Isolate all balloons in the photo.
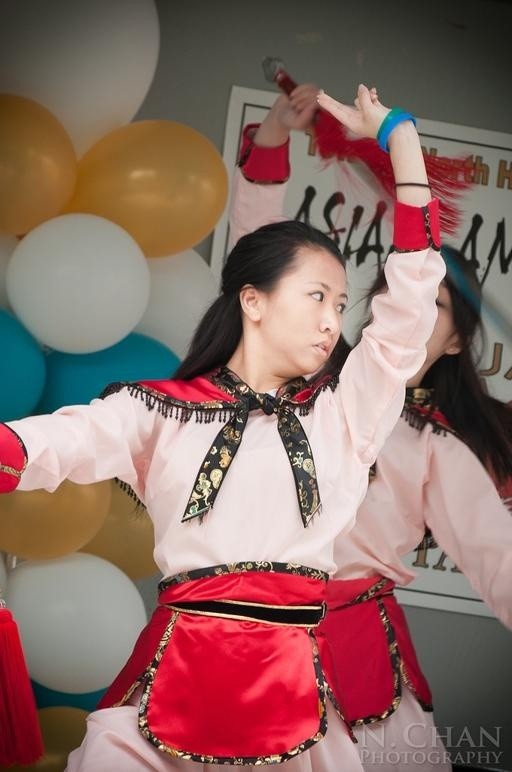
[0,1,228,772]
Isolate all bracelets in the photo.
[379,112,416,153]
[375,106,405,142]
[391,179,432,191]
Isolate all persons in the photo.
[0,81,449,772]
[225,81,512,772]
